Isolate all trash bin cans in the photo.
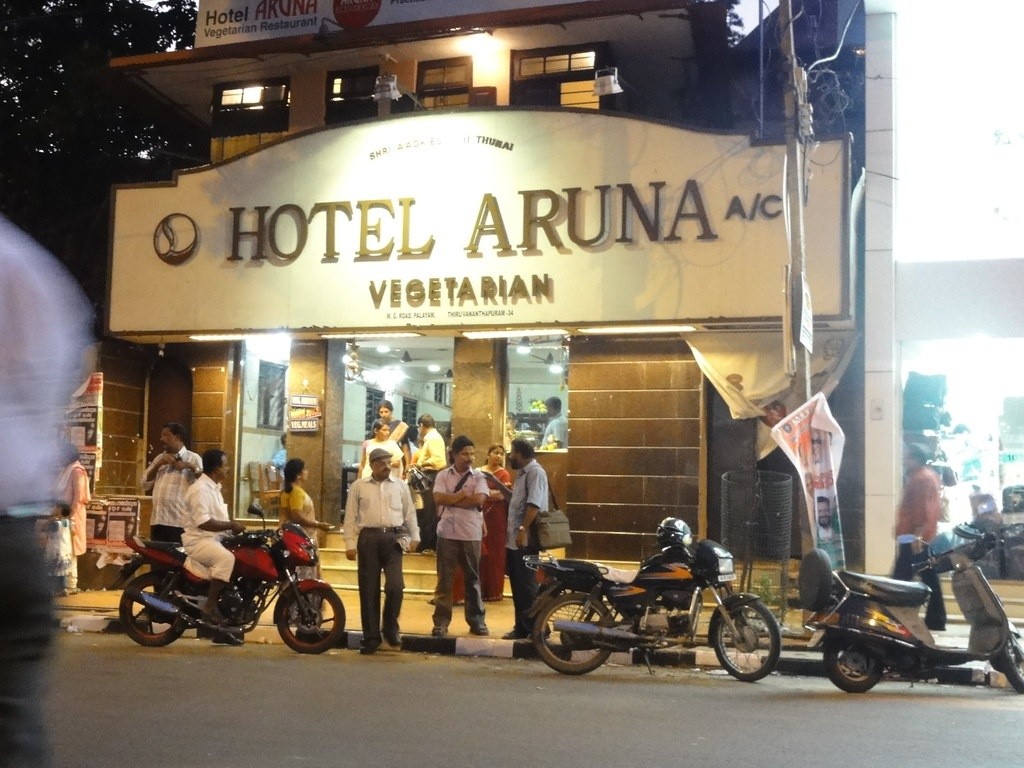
[973,521,1024,582]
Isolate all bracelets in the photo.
[316,521,318,526]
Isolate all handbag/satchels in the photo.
[525,465,572,550]
[425,515,442,551]
[408,467,430,492]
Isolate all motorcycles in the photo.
[522,524,783,682]
[108,504,347,655]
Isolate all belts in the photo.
[362,528,396,533]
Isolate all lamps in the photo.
[594,66,623,95]
[372,73,428,112]
[513,337,566,373]
[344,342,454,385]
[312,17,352,39]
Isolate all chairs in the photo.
[246,461,283,515]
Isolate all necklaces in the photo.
[500,484,506,492]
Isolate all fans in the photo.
[530,351,561,365]
[386,350,431,365]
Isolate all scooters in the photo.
[797,491,1024,694]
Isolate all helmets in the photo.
[656,517,692,547]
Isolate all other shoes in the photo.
[470,622,490,637]
[432,623,447,637]
[422,548,436,556]
[526,624,550,640]
[54,589,70,598]
[502,628,527,639]
[67,587,77,594]
[382,630,403,648]
[360,634,383,654]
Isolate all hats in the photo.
[370,448,393,465]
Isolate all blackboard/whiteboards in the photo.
[85,499,109,544]
[106,498,140,543]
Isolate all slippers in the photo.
[212,632,244,645]
[199,606,230,626]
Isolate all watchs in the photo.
[518,525,528,531]
[191,466,199,475]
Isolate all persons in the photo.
[271,433,287,485]
[521,419,534,431]
[508,412,518,432]
[892,443,947,632]
[181,448,249,649]
[432,434,490,636]
[539,396,569,449]
[356,400,423,485]
[0,214,93,768]
[344,448,422,656]
[536,419,542,432]
[817,495,832,540]
[481,439,548,640]
[140,420,204,593]
[474,442,513,601]
[50,442,91,597]
[37,501,76,598]
[278,458,335,635]
[408,413,448,557]
